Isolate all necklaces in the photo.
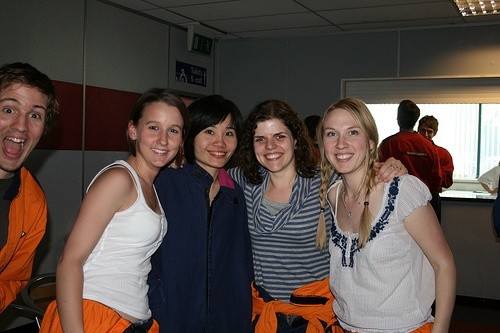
[340,189,367,219]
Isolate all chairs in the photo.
[20,273,56,313]
[0,305,49,333]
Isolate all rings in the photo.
[391,165,395,170]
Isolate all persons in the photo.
[311,95,458,333]
[158,101,411,333]
[375,97,442,224]
[145,92,257,333]
[35,86,192,333]
[414,113,455,224]
[1,60,64,325]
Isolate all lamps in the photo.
[452,0,500,18]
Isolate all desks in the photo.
[435,190,500,306]
